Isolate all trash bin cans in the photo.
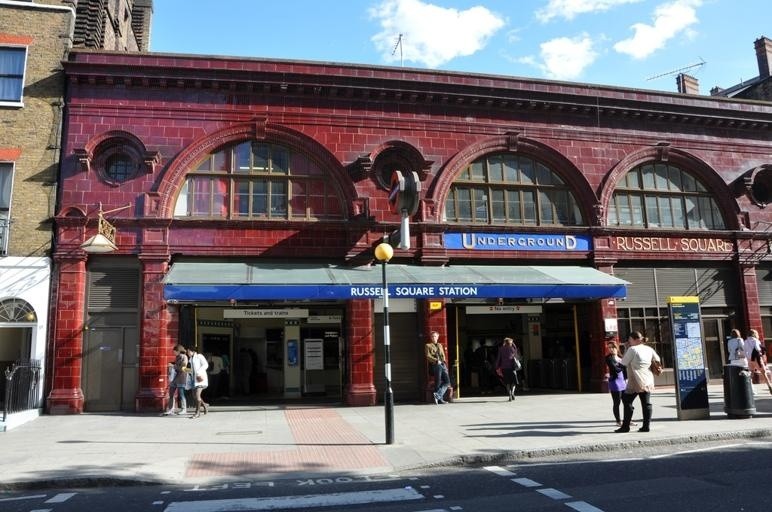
[724,365,756,419]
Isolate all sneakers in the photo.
[433,392,448,404]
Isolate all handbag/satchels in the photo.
[652,358,663,375]
[735,338,745,359]
[513,357,522,371]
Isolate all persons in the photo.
[744,329,772,395]
[605,342,638,426]
[495,337,519,401]
[728,328,756,395]
[163,344,257,419]
[610,331,661,433]
[425,331,450,404]
[472,340,496,394]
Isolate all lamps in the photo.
[77,201,133,253]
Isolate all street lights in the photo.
[374,243,394,444]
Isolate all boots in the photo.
[614,406,638,433]
[639,405,652,432]
[162,398,175,415]
[507,384,515,401]
[190,400,209,419]
[177,399,187,415]
